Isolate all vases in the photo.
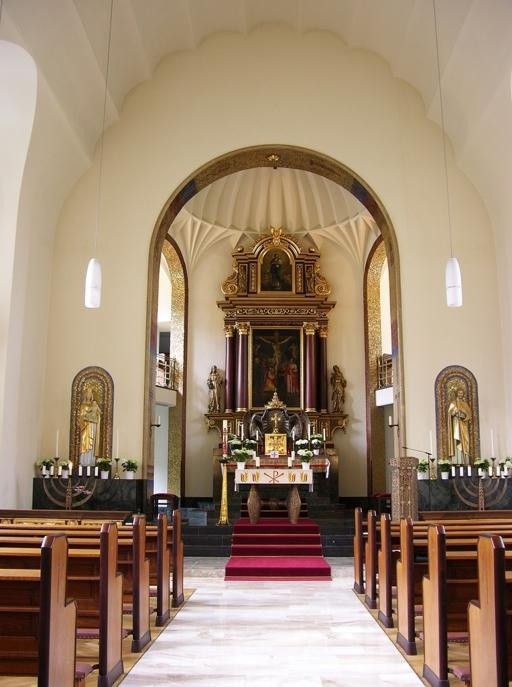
[101,471,108,479]
[44,470,50,478]
[61,470,69,479]
[246,450,254,455]
[481,469,488,477]
[236,462,246,469]
[314,449,320,456]
[298,449,305,456]
[231,450,236,455]
[418,472,427,480]
[441,472,449,480]
[301,462,310,470]
[125,471,134,479]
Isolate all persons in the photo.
[77,390,102,465]
[206,364,224,412]
[329,365,347,412]
[446,387,475,467]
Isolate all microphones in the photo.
[402,447,409,449]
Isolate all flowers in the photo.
[474,457,490,472]
[245,439,257,450]
[297,448,313,463]
[121,457,139,472]
[233,448,248,462]
[310,433,324,449]
[416,456,429,472]
[228,439,242,450]
[438,458,451,472]
[96,457,113,471]
[57,456,71,470]
[295,439,308,449]
[36,456,56,470]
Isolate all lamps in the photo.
[433,0,463,307]
[84,0,113,308]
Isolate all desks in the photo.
[227,460,326,518]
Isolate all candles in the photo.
[490,429,495,458]
[291,451,295,461]
[157,416,160,424]
[496,467,501,476]
[241,424,243,441]
[58,466,62,475]
[323,429,326,441]
[478,468,481,476]
[238,422,240,436]
[79,465,83,476]
[452,466,455,477]
[256,457,260,467]
[256,430,258,440]
[69,463,73,475]
[467,466,471,476]
[87,466,91,476]
[459,467,464,476]
[287,457,292,467]
[389,416,391,425]
[292,429,294,440]
[95,467,98,476]
[42,466,46,475]
[504,466,508,475]
[307,424,310,440]
[430,430,432,458]
[50,466,55,475]
[252,451,256,460]
[488,466,493,476]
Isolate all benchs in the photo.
[0,510,185,687]
[351,507,512,687]
